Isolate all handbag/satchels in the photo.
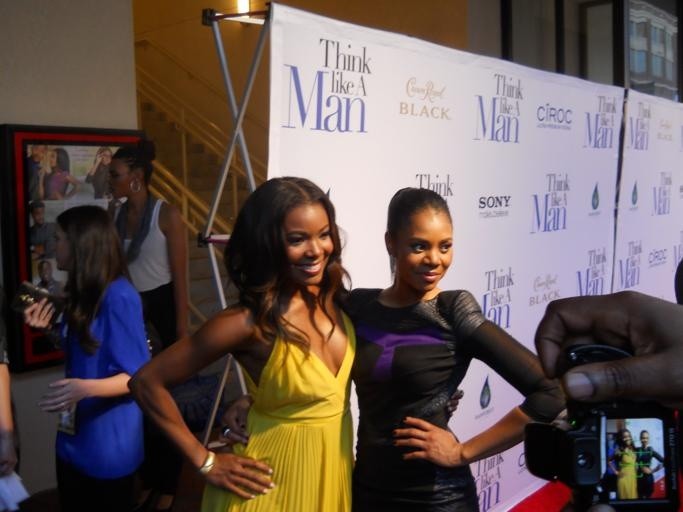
[171,375,225,429]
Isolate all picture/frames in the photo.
[1,124,145,374]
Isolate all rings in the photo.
[222,425,231,436]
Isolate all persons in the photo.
[104,138,189,510]
[127,178,358,511]
[0,313,32,512]
[607,439,619,490]
[534,286,682,512]
[22,204,155,512]
[608,428,640,499]
[635,430,664,498]
[216,187,565,512]
[27,143,113,302]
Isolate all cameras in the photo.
[525,343,679,512]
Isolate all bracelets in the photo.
[196,448,216,475]
